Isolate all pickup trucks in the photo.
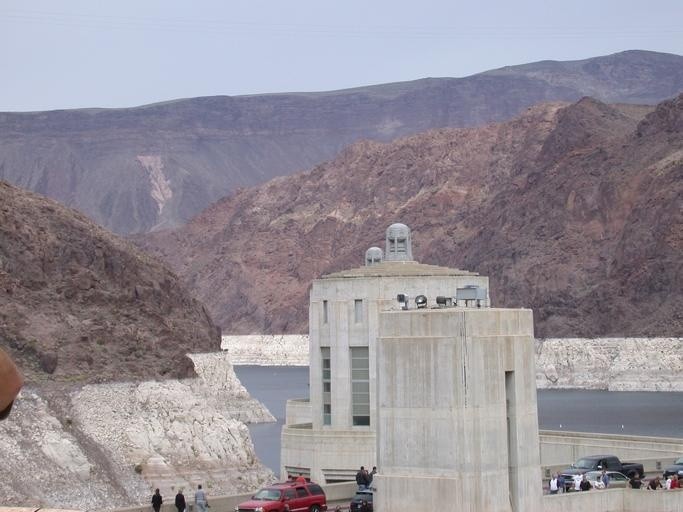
[556,454,646,490]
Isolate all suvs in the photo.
[232,481,328,512]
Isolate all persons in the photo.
[355,466,368,489]
[193,485,211,512]
[174,489,187,512]
[367,466,377,482]
[152,488,163,512]
[647,476,663,490]
[548,471,593,495]
[294,472,307,482]
[594,476,606,490]
[662,473,683,490]
[601,469,610,488]
[286,475,292,482]
[625,472,646,490]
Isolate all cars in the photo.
[348,489,374,511]
[662,456,682,483]
[566,471,641,490]
